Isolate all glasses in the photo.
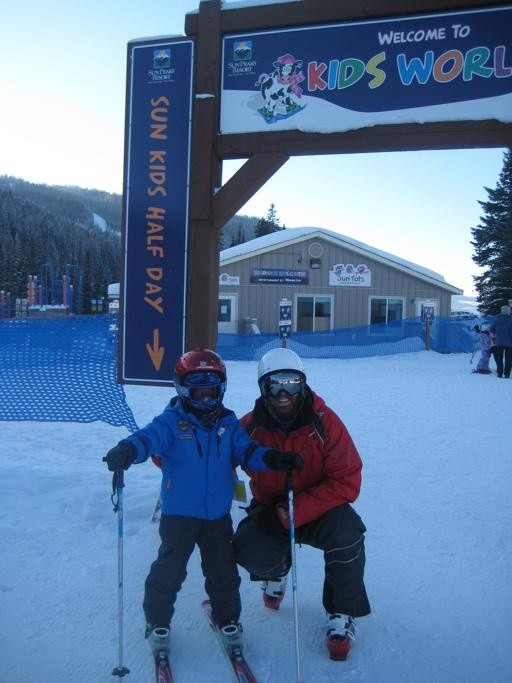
[181,372,224,387]
[263,383,301,397]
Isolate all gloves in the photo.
[264,449,305,473]
[255,508,286,537]
[105,442,137,472]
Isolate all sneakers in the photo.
[260,574,288,598]
[325,612,357,645]
[143,622,172,653]
[211,618,244,648]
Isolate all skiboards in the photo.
[144,600,257,683]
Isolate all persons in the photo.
[468,305,511,378]
[105,344,305,665]
[226,345,367,656]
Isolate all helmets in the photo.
[172,347,228,420]
[256,347,310,429]
